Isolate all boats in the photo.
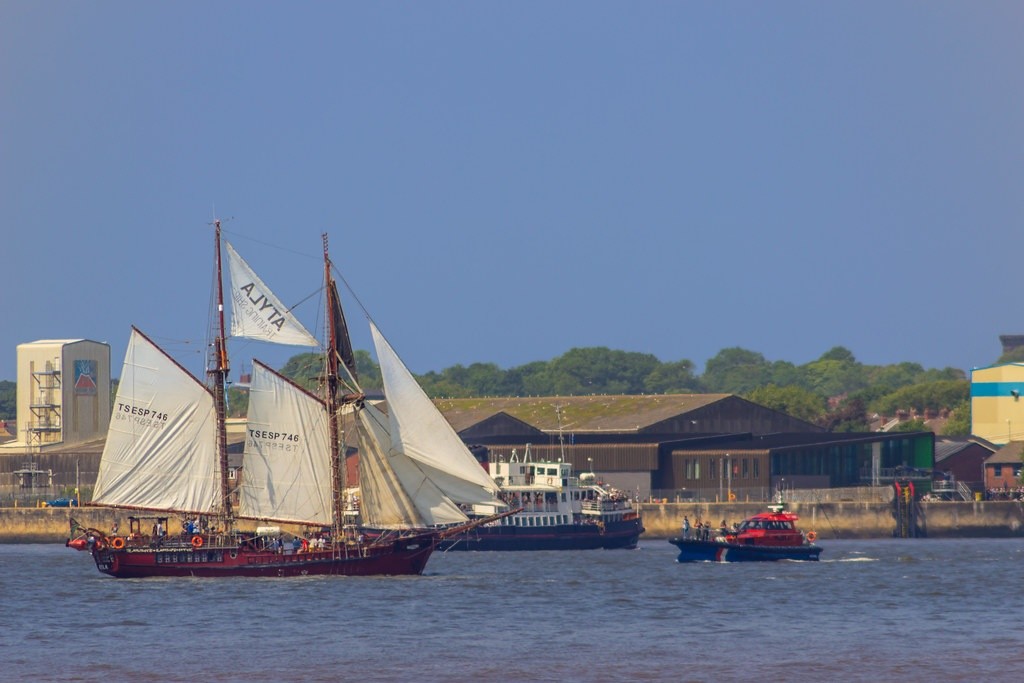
[667,475,824,565]
[341,403,646,553]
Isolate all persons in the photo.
[694,520,711,541]
[985,486,1024,500]
[332,531,364,545]
[181,518,196,536]
[276,533,286,556]
[302,537,307,552]
[308,534,326,553]
[682,515,691,538]
[741,520,786,530]
[718,519,738,540]
[203,519,217,534]
[110,522,120,538]
[152,520,165,550]
[489,469,631,525]
[292,535,300,551]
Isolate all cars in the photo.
[46,497,78,507]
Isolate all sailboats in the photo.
[64,215,526,580]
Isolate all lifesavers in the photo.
[547,478,553,485]
[112,537,125,549]
[806,531,817,542]
[192,536,203,547]
[729,494,736,500]
